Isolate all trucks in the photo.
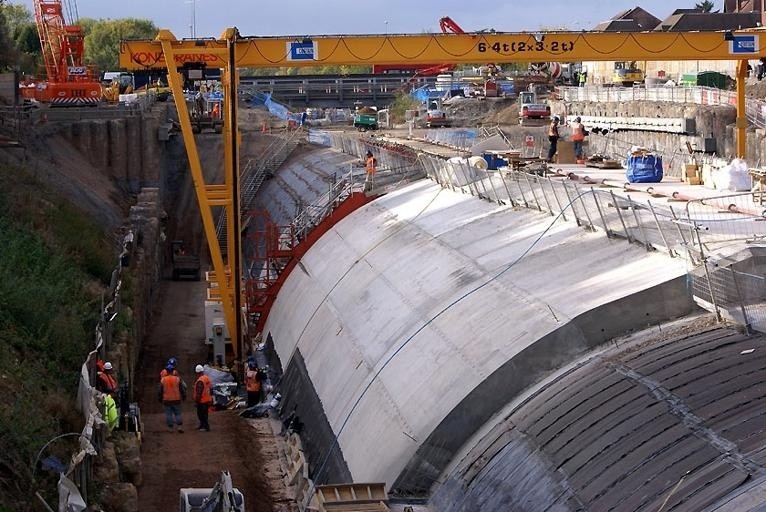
[353,113,378,131]
[170,237,202,281]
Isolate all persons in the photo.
[572,117,585,162]
[96,358,212,432]
[211,104,219,121]
[757,60,764,81]
[579,73,586,87]
[747,62,752,78]
[196,93,206,116]
[546,117,560,162]
[363,150,377,192]
[244,357,258,385]
[246,361,267,407]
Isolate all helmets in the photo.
[248,361,258,369]
[103,362,112,369]
[195,365,204,372]
[166,358,177,370]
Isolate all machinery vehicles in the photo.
[197,267,240,372]
[602,60,645,88]
[190,89,225,135]
[525,61,574,89]
[15,1,104,110]
[181,471,245,512]
[517,92,552,130]
[414,94,456,128]
[105,77,173,105]
